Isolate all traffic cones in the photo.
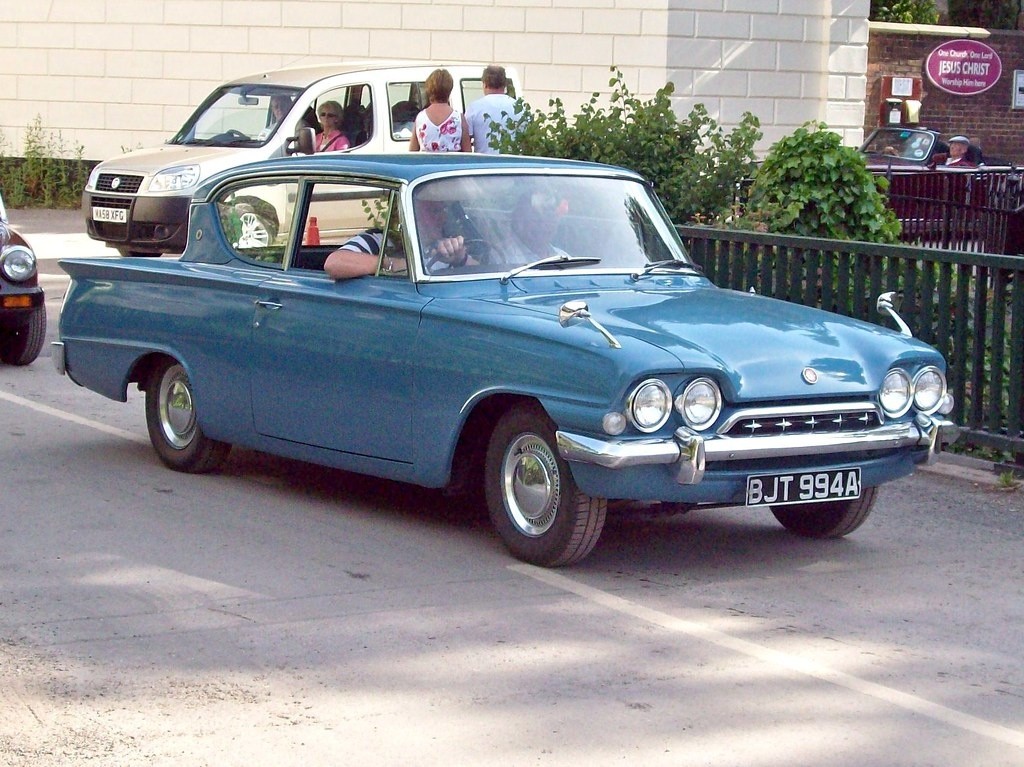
[305,216,321,246]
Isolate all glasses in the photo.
[320,113,336,117]
[429,205,449,217]
[272,103,286,108]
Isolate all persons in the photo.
[944,135,980,167]
[409,68,472,152]
[322,192,482,280]
[885,126,946,163]
[465,63,527,155]
[255,95,293,142]
[485,185,575,266]
[311,100,351,153]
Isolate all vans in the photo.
[81,56,524,257]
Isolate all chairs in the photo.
[302,106,323,135]
[337,106,368,149]
[928,141,952,165]
[965,145,983,167]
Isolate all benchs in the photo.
[233,245,345,271]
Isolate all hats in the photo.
[948,135,970,145]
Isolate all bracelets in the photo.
[387,257,394,272]
[453,253,469,268]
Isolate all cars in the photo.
[49,153,960,569]
[0,190,47,365]
[723,124,1024,231]
